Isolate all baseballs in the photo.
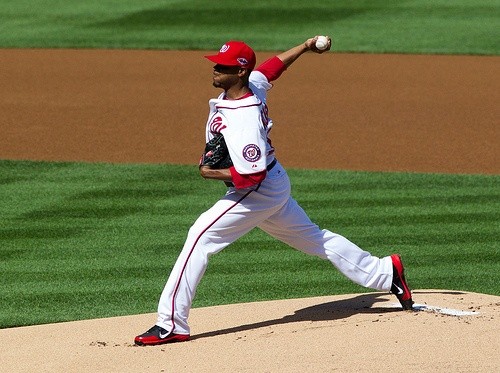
[315,35,328,50]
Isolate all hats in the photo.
[204,40,256,69]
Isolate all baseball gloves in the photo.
[198,134,233,188]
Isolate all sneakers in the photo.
[134,326,190,345]
[391,254,414,310]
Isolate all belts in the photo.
[267,158,277,172]
